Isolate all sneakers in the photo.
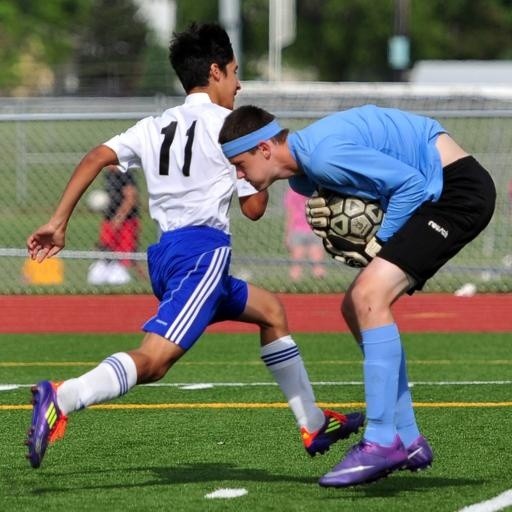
[301,410,433,487]
[26,380,66,467]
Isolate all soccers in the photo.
[325,196,384,250]
[86,190,111,213]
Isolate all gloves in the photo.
[305,190,332,238]
[322,236,382,267]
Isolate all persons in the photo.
[87,164,141,286]
[218,104,495,487]
[20,256,63,286]
[283,188,324,280]
[26,22,366,469]
